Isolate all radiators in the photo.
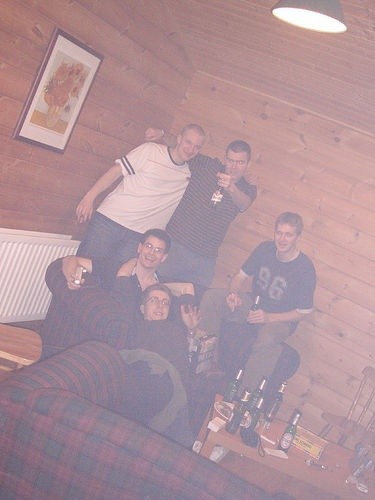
[0,228,81,322]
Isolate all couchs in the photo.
[44,256,301,407]
[0,340,290,500]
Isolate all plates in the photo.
[214,401,251,426]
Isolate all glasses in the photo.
[146,296,170,306]
[142,243,163,255]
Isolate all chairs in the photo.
[0,323,44,368]
[319,366,375,452]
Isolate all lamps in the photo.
[272,0,348,34]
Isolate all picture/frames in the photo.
[14,27,103,152]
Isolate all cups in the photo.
[72,264,87,286]
[351,459,373,477]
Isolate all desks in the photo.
[199,391,375,500]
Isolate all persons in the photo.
[75,122,257,306]
[61,229,202,449]
[198,213,316,401]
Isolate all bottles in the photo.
[247,296,260,325]
[225,389,252,434]
[263,382,288,421]
[240,394,265,437]
[277,413,301,454]
[207,186,224,210]
[242,377,267,414]
[223,368,244,403]
